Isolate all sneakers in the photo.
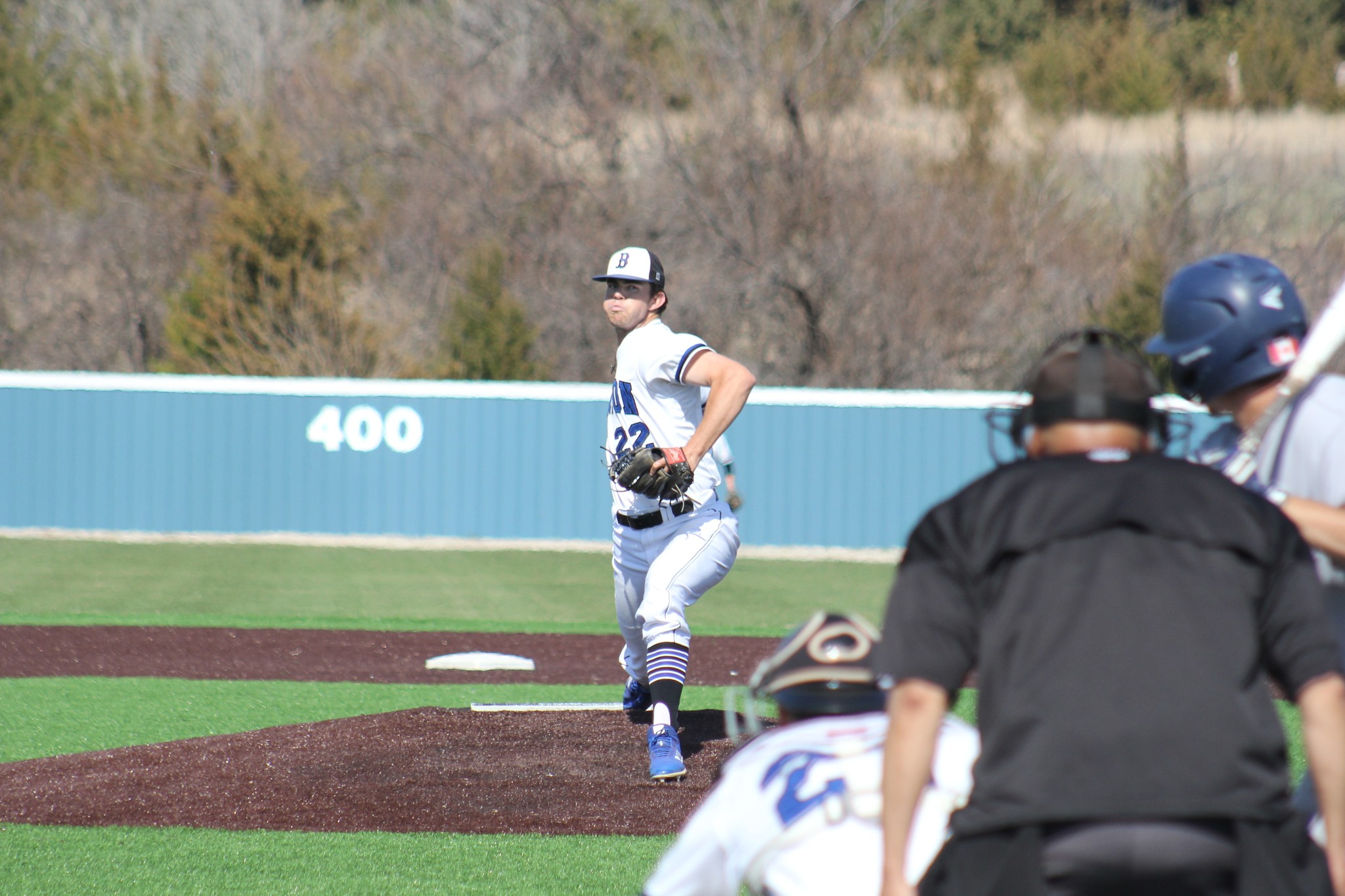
[647,723,686,782]
[623,676,652,714]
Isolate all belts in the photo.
[617,499,694,530]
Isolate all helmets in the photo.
[751,614,883,714]
[1142,254,1307,399]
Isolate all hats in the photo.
[592,246,665,286]
[1010,350,1151,445]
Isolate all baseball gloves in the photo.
[607,438,694,498]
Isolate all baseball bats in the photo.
[1241,277,1345,459]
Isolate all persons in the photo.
[1142,254,1345,854]
[642,614,980,896]
[593,247,756,782]
[874,330,1345,896]
